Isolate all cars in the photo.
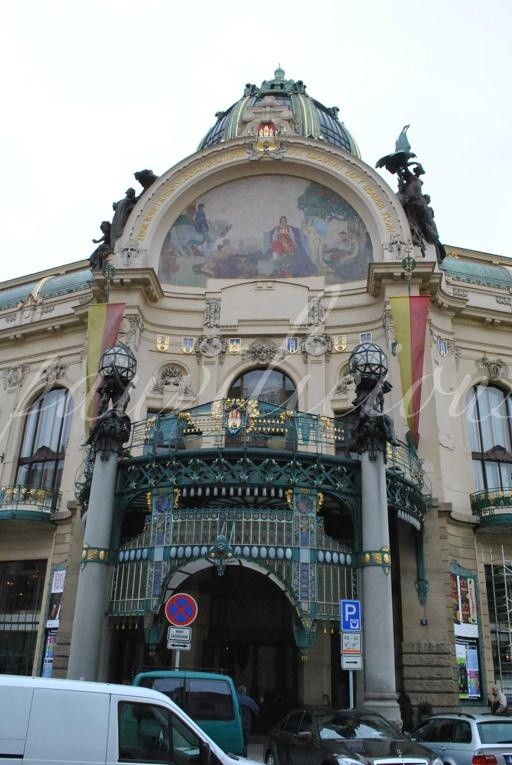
[405,711,512,765]
[263,704,445,764]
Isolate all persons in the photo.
[237,685,260,750]
[491,685,508,714]
[192,201,374,282]
[395,689,414,735]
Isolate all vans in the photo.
[0,673,268,765]
[119,670,246,759]
